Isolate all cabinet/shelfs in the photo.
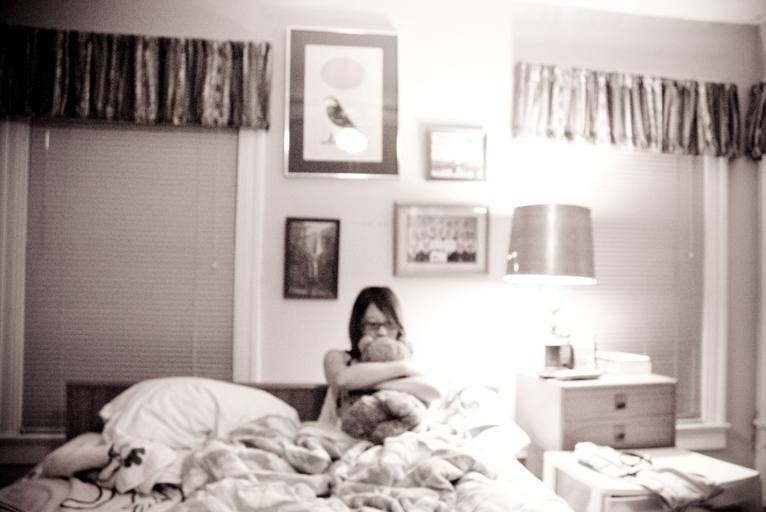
[541,447,763,511]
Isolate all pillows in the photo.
[99,375,298,443]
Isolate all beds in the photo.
[0,383,574,512]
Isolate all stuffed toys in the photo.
[339,333,426,439]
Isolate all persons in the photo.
[315,286,444,452]
[402,218,479,268]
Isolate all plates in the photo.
[536,367,602,379]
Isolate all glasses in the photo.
[366,321,398,330]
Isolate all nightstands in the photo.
[519,368,677,450]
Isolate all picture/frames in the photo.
[283,216,341,301]
[394,201,490,280]
[284,25,400,178]
[427,127,487,181]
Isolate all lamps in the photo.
[503,203,604,380]
